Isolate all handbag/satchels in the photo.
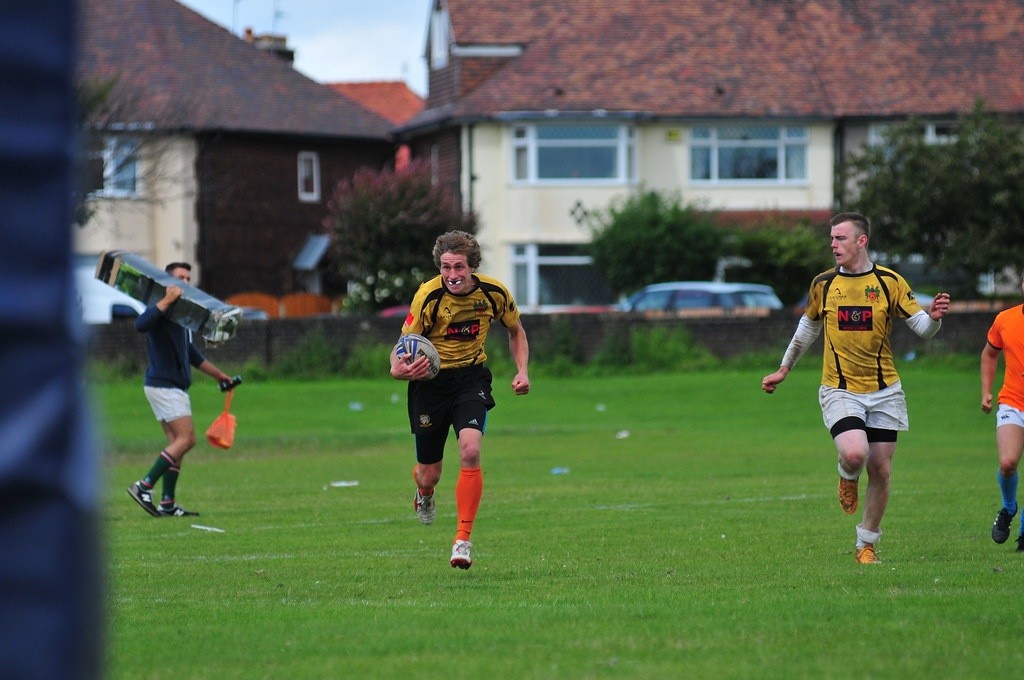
[206,388,237,449]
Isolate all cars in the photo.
[73,261,151,325]
[612,280,785,316]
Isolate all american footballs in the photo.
[397,333,441,381]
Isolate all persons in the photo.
[389,231,529,569]
[127,262,233,518]
[981,272,1024,553]
[762,212,950,563]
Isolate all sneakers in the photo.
[126,480,161,517]
[1015,531,1024,553]
[413,488,437,525]
[856,544,881,565]
[992,501,1018,544]
[450,536,473,570]
[838,475,859,515]
[157,504,199,517]
